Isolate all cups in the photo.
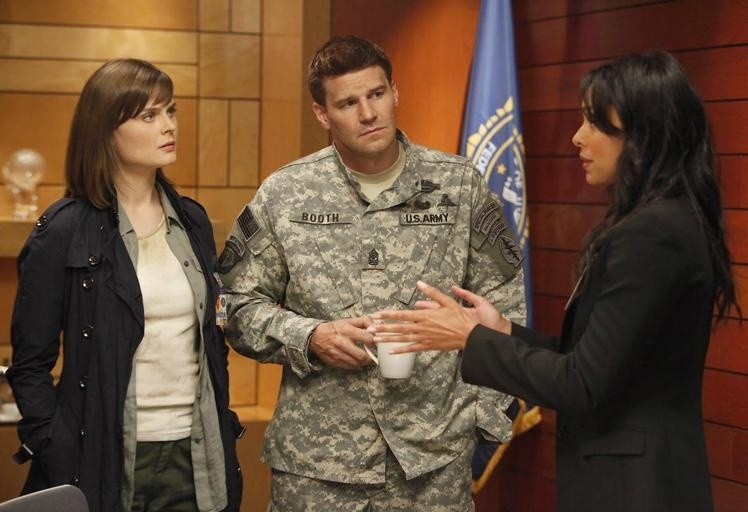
[364,332,415,380]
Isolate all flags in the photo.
[460,0,542,494]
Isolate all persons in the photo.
[5,58,247,512]
[367,50,743,511]
[213,36,528,512]
